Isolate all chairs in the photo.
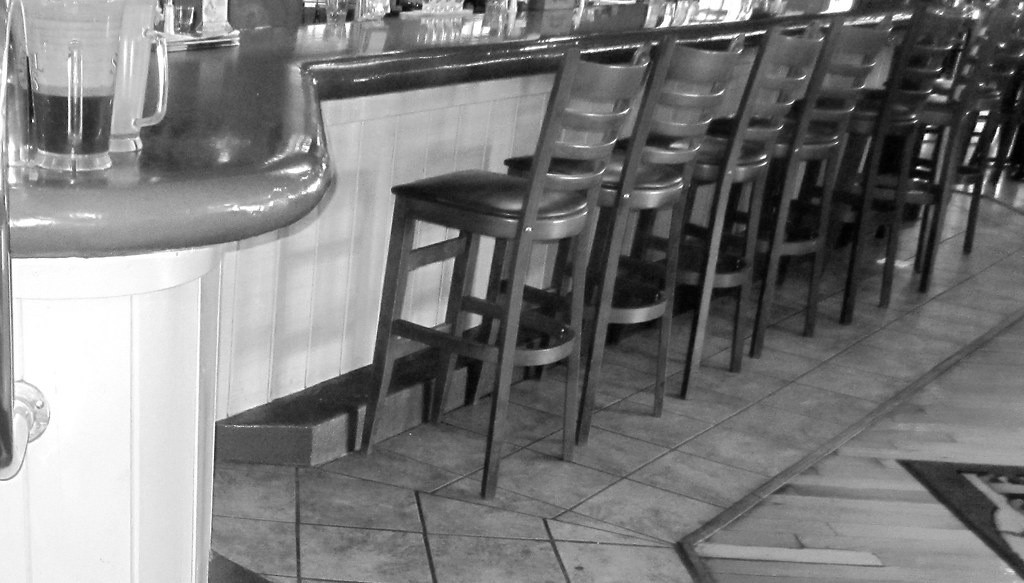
[362,0,1024,499]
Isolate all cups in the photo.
[643,0,788,30]
[0,0,195,172]
[323,1,530,31]
